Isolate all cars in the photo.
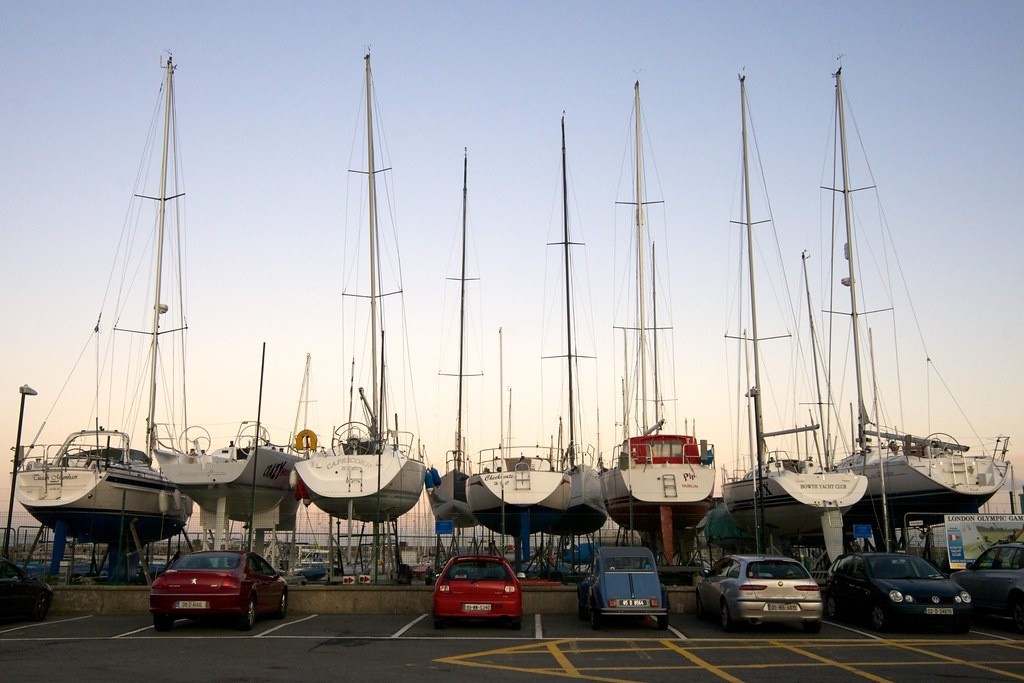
[0,556,52,626]
[149,550,287,631]
[698,553,823,635]
[430,556,523,629]
[580,547,670,632]
[827,552,973,634]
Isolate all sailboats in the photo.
[26,54,199,546]
[810,58,1011,532]
[421,145,497,529]
[535,112,606,540]
[700,74,872,544]
[305,45,419,527]
[598,76,718,536]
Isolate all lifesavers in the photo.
[296,429,318,451]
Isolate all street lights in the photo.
[3,384,37,559]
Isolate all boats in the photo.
[467,440,575,538]
[152,414,309,519]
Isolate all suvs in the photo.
[952,541,1024,635]
